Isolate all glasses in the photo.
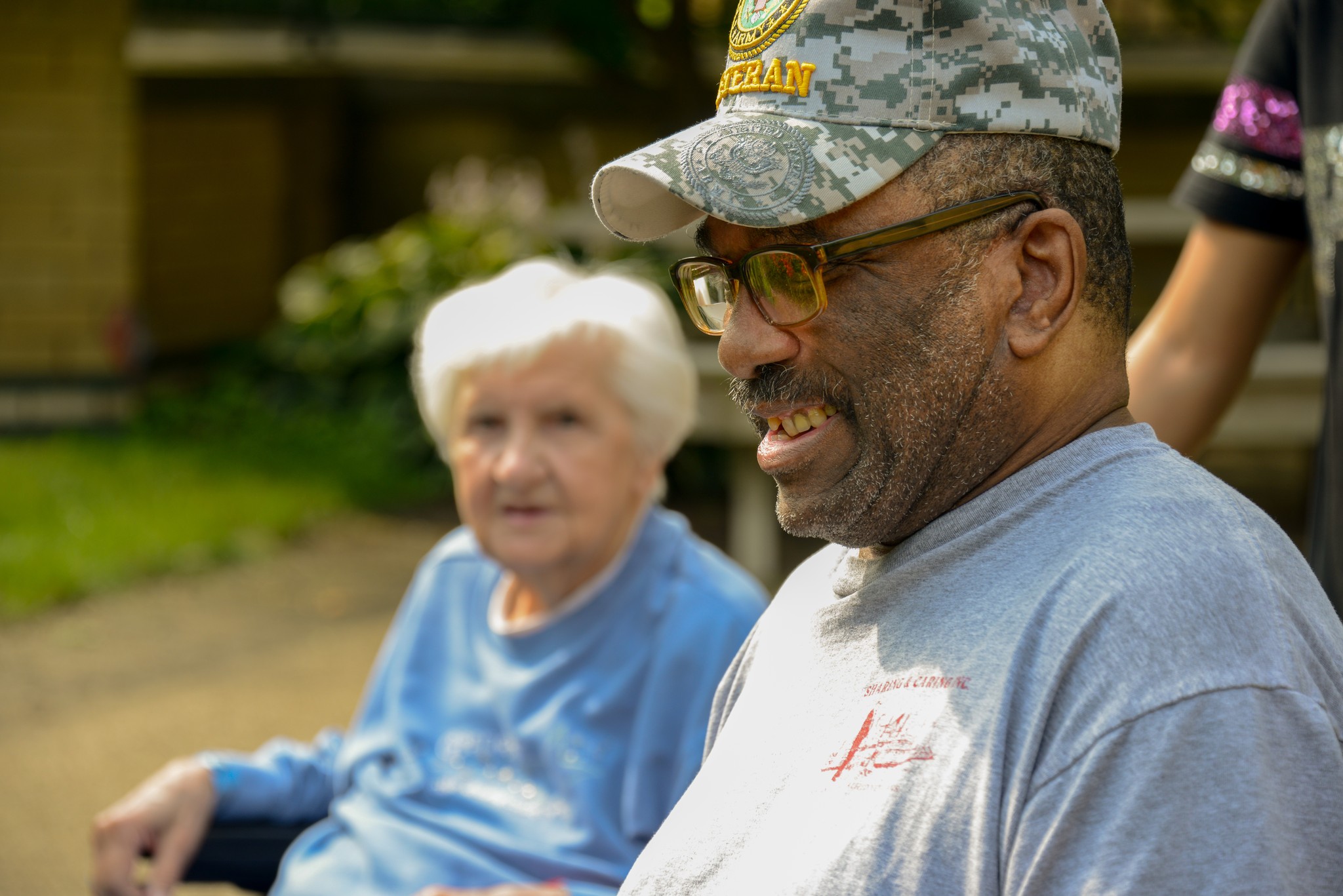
[669,190,1047,336]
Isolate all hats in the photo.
[588,0,1123,245]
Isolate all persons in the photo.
[89,255,770,896]
[1125,0,1343,624]
[590,1,1343,896]
